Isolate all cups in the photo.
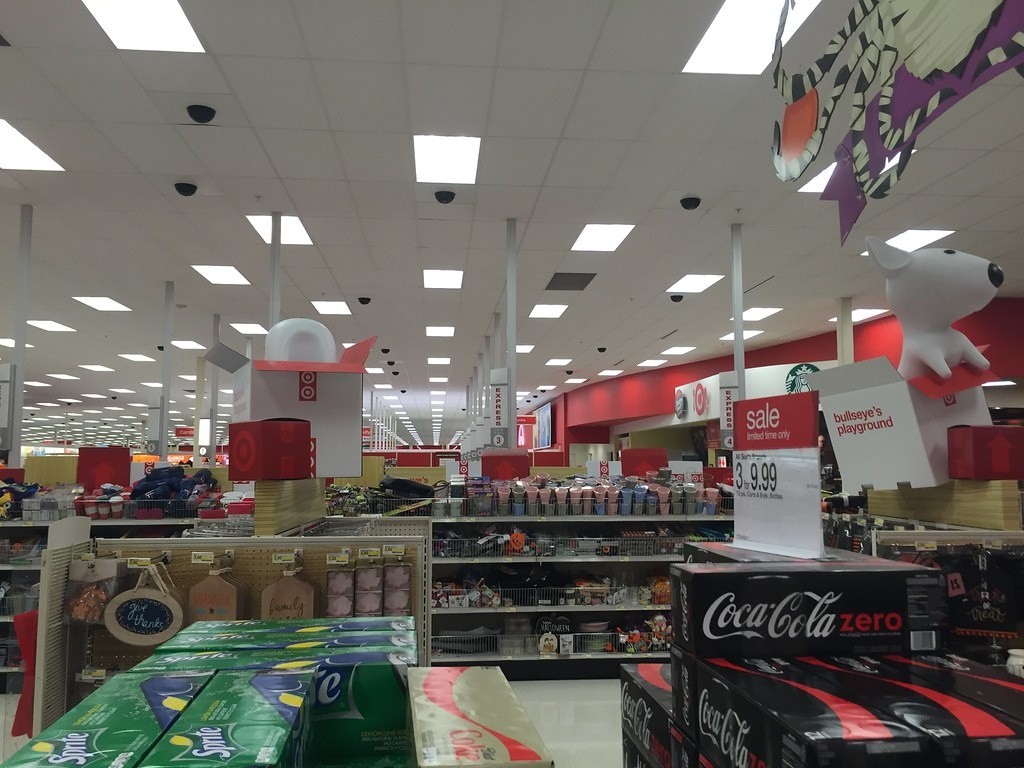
[494,466,722,517]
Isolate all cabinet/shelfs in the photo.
[0,477,736,696]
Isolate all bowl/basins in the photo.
[577,620,611,632]
[580,638,613,651]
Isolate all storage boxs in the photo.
[0,669,218,768]
[683,542,820,564]
[793,666,1024,768]
[620,663,673,768]
[225,417,311,482]
[947,424,1024,481]
[885,652,1024,722]
[126,646,417,759]
[671,565,948,658]
[816,546,897,564]
[694,661,931,768]
[136,669,316,768]
[405,666,556,768]
[622,728,653,768]
[802,343,1001,496]
[177,615,415,635]
[202,335,377,479]
[155,630,418,653]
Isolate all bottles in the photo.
[1006,649,1024,678]
